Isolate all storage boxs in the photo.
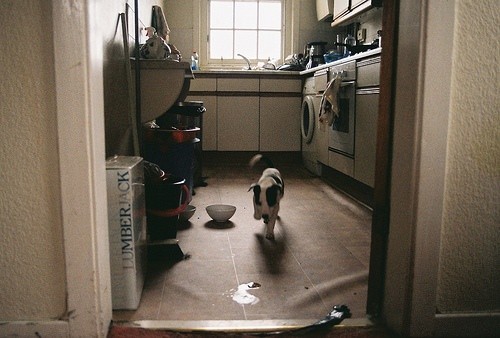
[106,155,151,313]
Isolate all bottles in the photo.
[191,51,199,71]
[337,33,344,52]
[343,34,356,57]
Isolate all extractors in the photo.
[330,1,384,28]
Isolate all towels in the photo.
[317,74,341,126]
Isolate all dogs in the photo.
[247,168,284,239]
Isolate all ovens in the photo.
[322,60,356,196]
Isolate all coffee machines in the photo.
[304,41,327,67]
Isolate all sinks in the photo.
[205,66,246,72]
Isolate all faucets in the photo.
[237,53,252,70]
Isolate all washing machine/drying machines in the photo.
[299,77,322,176]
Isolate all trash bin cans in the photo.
[175,101,209,187]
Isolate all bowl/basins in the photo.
[170,125,199,143]
[179,205,196,224]
[323,52,342,63]
[206,204,236,223]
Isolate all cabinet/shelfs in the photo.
[330,0,384,30]
[188,54,380,211]
